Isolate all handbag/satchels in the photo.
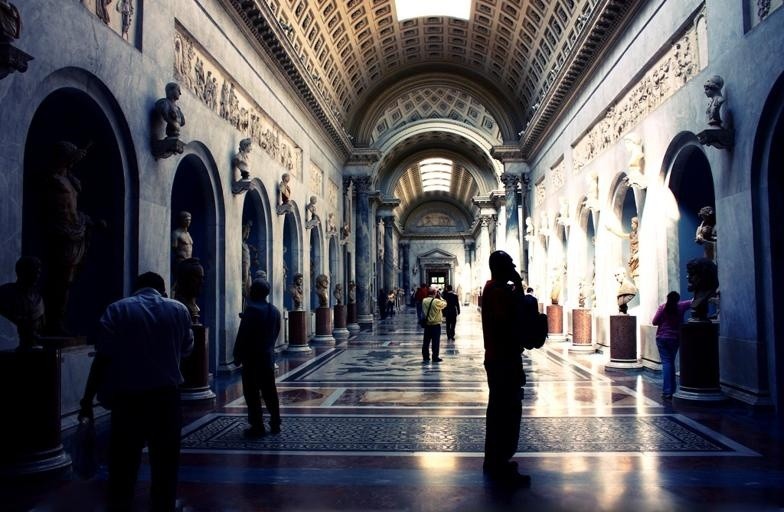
[421,312,427,327]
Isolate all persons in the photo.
[376,278,462,365]
[155,81,186,146]
[236,138,349,245]
[700,73,728,131]
[481,249,548,489]
[229,279,282,441]
[46,136,106,328]
[172,209,203,324]
[242,219,260,297]
[611,267,637,311]
[603,216,637,277]
[683,257,719,317]
[651,292,698,401]
[172,34,295,170]
[687,202,715,244]
[288,271,357,310]
[3,256,46,357]
[78,272,194,510]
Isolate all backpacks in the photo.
[523,295,548,350]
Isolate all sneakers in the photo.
[270,423,281,434]
[433,358,443,362]
[509,462,518,468]
[519,474,532,483]
[244,424,266,437]
